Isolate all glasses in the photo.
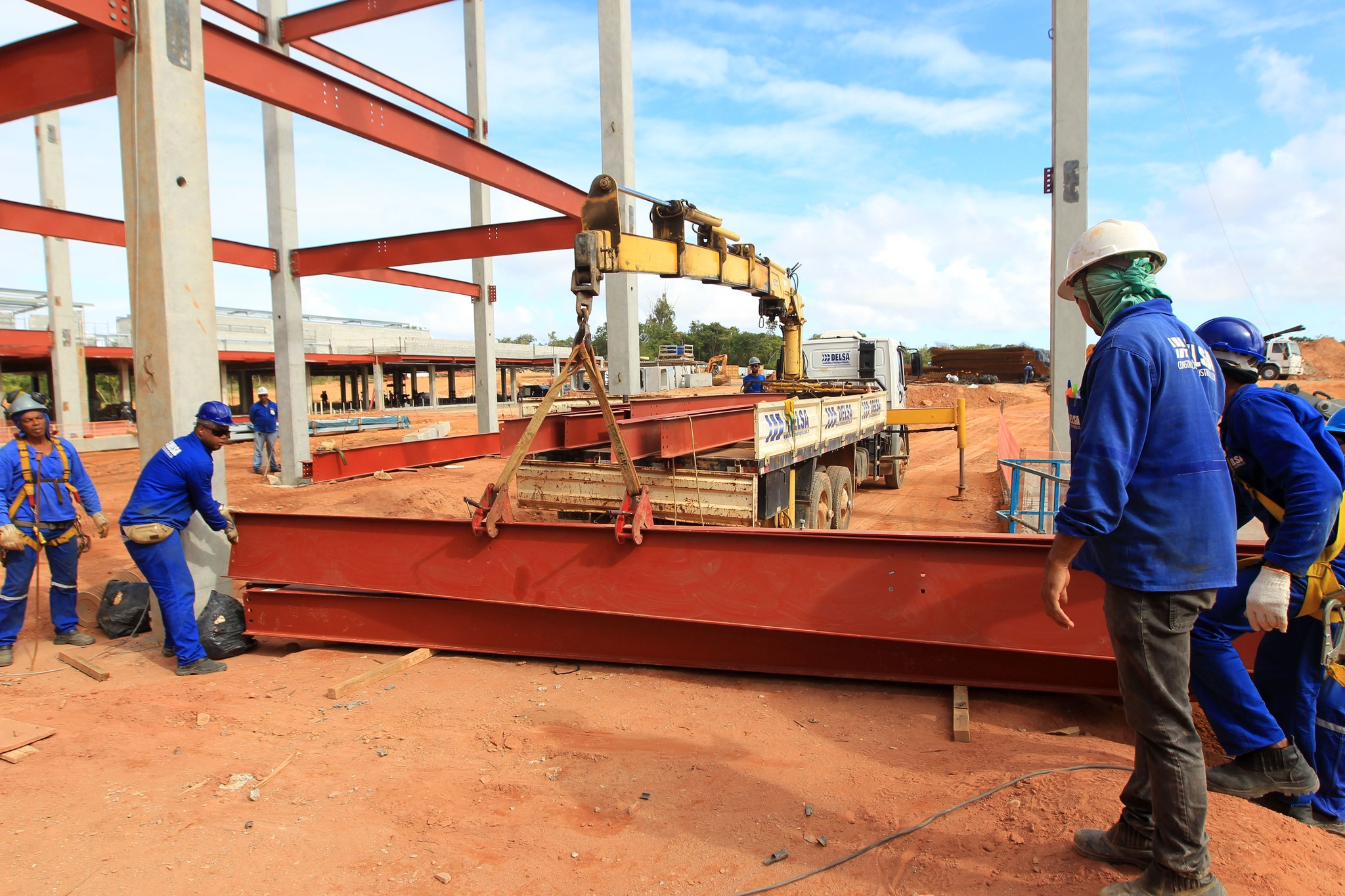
[751,365,759,368]
[203,426,231,438]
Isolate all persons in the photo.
[117,401,238,675]
[320,391,328,406]
[1023,366,1034,384]
[743,357,772,393]
[403,394,410,399]
[1041,220,1238,896]
[249,386,281,473]
[384,390,393,407]
[0,389,109,666]
[1189,317,1345,834]
[419,392,424,399]
[361,392,363,400]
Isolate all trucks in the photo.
[1256,338,1304,381]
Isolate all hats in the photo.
[1214,349,1259,386]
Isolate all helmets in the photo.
[4,392,50,419]
[1056,217,1167,301]
[749,356,761,365]
[194,400,239,426]
[1194,317,1266,362]
[256,386,268,394]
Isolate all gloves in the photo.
[217,503,234,524]
[1243,565,1291,633]
[91,510,108,539]
[222,519,238,545]
[0,524,24,552]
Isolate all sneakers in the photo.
[1206,734,1320,800]
[162,646,176,657]
[1099,866,1228,896]
[1074,826,1152,870]
[254,467,264,474]
[270,468,282,472]
[53,629,95,646]
[0,645,13,666]
[1308,809,1345,836]
[1251,794,1313,823]
[176,656,227,676]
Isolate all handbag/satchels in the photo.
[121,522,174,544]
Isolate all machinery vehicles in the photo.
[452,173,972,541]
